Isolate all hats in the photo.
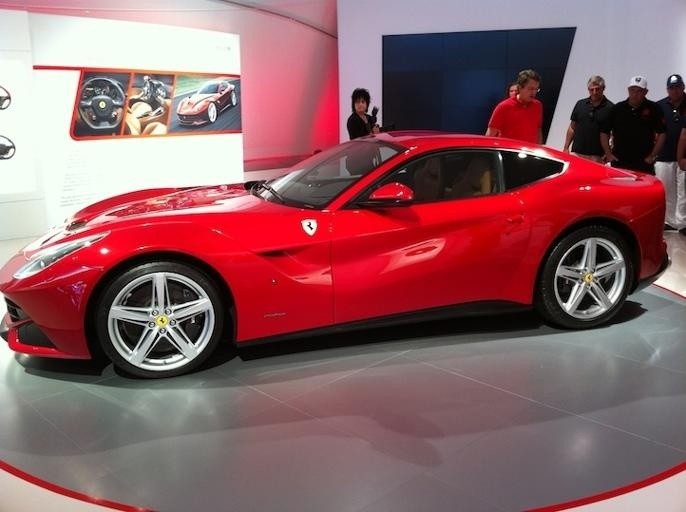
[628,76,649,91]
[666,74,683,88]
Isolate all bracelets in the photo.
[604,149,612,154]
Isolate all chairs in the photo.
[412,154,493,203]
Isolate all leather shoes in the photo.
[663,223,678,230]
[679,227,685,238]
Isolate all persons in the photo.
[505,81,519,99]
[344,87,384,175]
[656,74,686,231]
[597,76,667,185]
[562,75,615,166]
[481,69,544,145]
[674,124,686,238]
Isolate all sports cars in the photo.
[176,79,237,126]
[0,131,672,379]
